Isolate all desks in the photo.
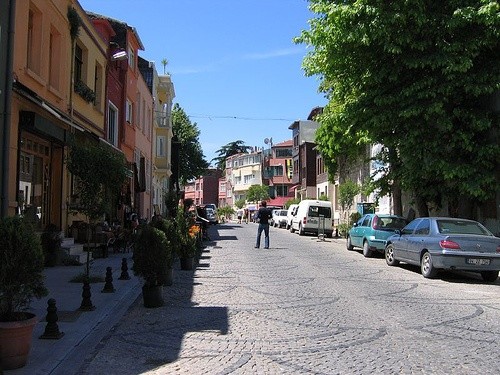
[94,231,127,258]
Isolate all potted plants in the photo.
[0,215,49,370]
[157,207,202,286]
[134,225,170,308]
[40,224,63,266]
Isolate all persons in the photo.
[253,201,273,249]
[237,208,243,224]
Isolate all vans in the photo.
[288,200,334,237]
[203,203,220,227]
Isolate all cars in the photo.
[272,204,299,229]
[384,216,500,282]
[345,213,410,257]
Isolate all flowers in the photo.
[188,225,201,239]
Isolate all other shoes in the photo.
[254,246,259,249]
[264,247,269,249]
